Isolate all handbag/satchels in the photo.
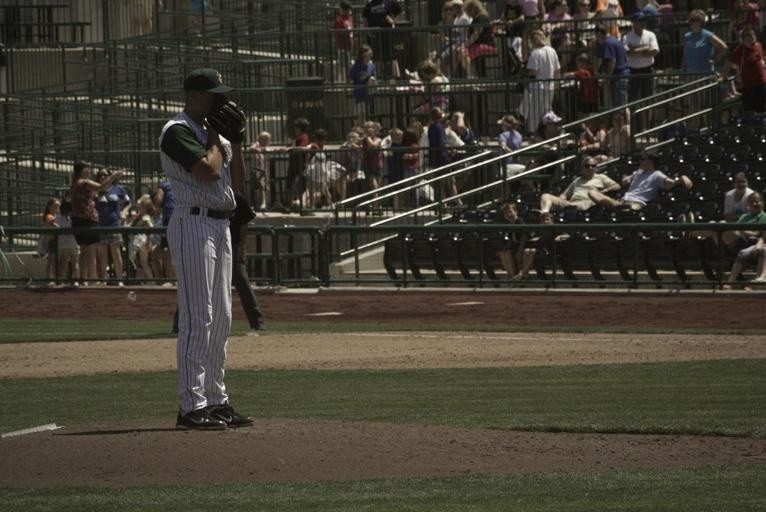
[417,179,435,202]
[59,198,75,213]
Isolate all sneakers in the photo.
[209,403,253,426]
[173,409,225,431]
[246,316,268,333]
[43,276,179,291]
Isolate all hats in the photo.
[425,105,443,119]
[72,160,90,169]
[180,68,234,94]
[494,110,562,128]
[631,11,647,21]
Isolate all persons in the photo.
[170,190,268,333]
[40,159,180,290]
[233,2,765,292]
[157,68,254,431]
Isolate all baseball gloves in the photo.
[207,96,247,143]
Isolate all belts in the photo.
[187,205,233,220]
